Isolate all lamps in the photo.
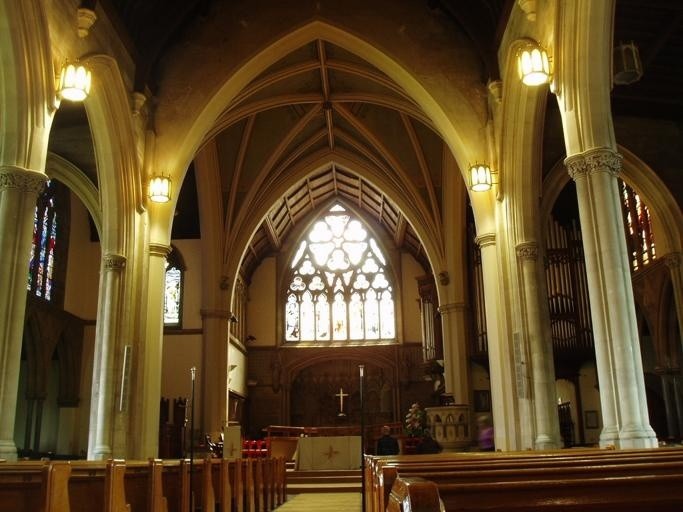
[516,46,554,87]
[148,172,172,203]
[614,40,642,87]
[468,160,498,193]
[56,58,94,104]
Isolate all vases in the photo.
[399,435,427,449]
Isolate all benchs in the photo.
[0,445,289,512]
[362,446,683,512]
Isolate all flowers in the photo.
[406,403,427,435]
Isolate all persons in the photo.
[473,414,495,452]
[375,424,401,456]
[413,432,443,453]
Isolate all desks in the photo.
[294,436,363,470]
